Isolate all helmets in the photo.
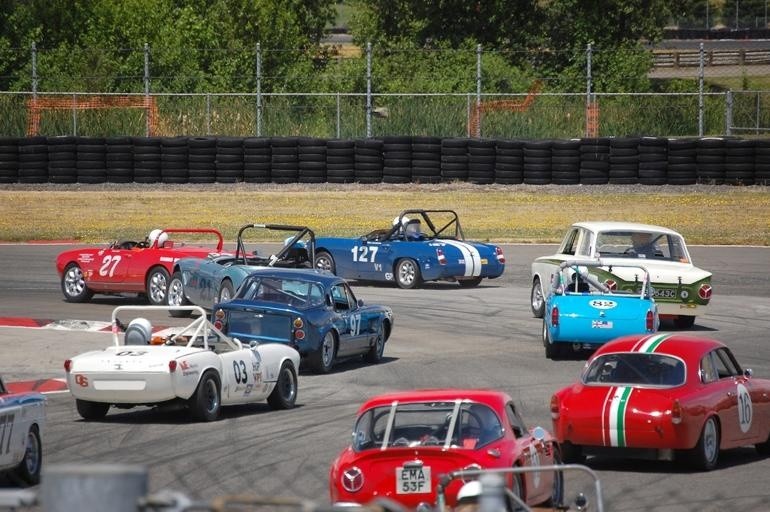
[567,265,587,284]
[392,216,411,236]
[127,318,152,341]
[284,236,304,249]
[466,406,497,432]
[631,232,654,249]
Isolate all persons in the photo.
[392,216,410,232]
[128,317,152,345]
[269,237,305,261]
[568,273,589,292]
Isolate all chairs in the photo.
[280,248,309,260]
[468,404,499,431]
[568,282,589,292]
